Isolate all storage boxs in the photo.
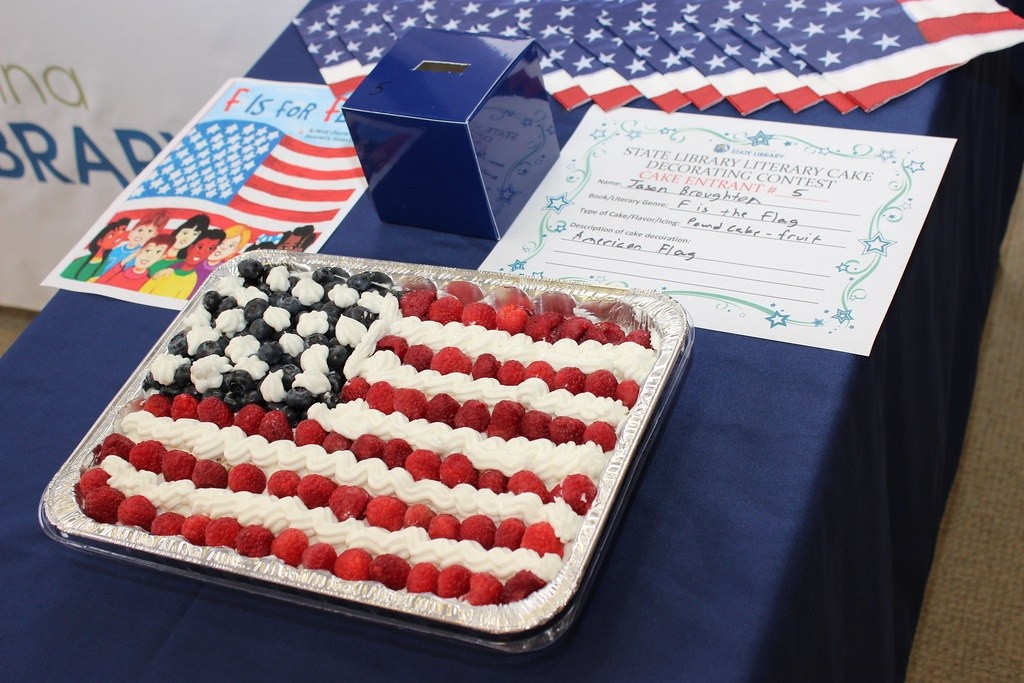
[341,29,562,240]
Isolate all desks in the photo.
[2,0,1023,683]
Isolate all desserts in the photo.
[80,257,657,604]
